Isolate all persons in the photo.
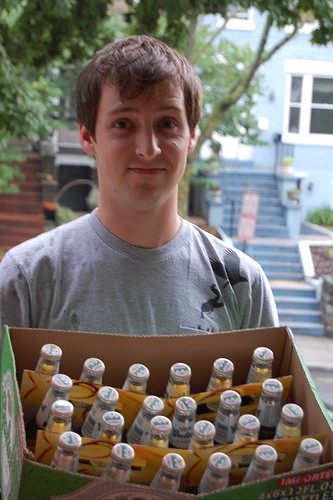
[0,35,280,347]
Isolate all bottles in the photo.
[32,343,324,493]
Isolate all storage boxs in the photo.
[0,325,333,500]
